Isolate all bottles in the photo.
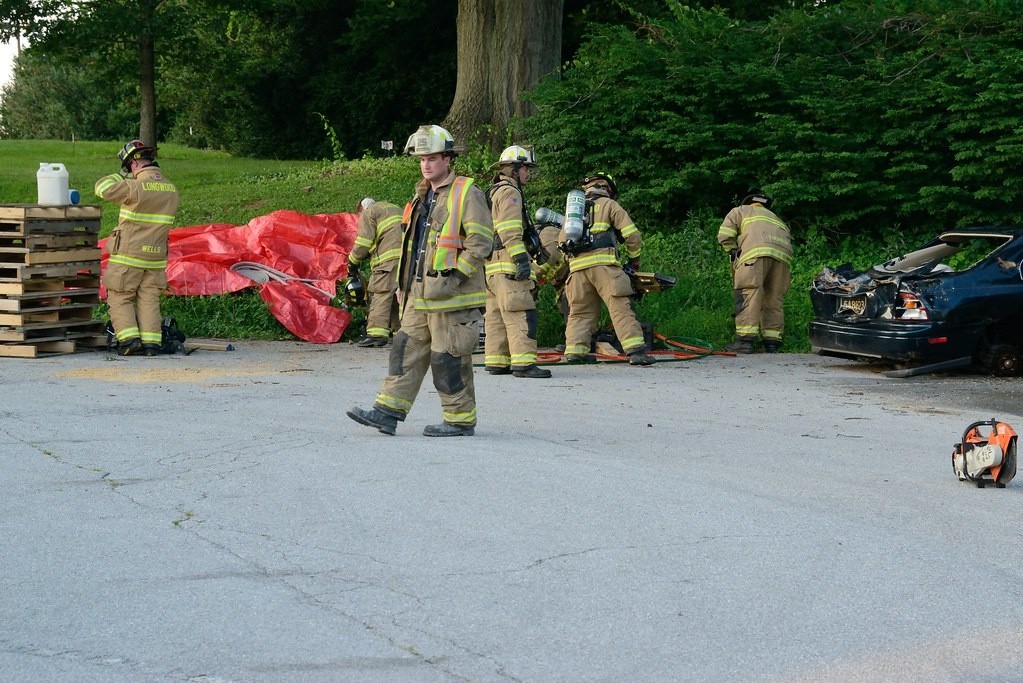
[36,162,69,207]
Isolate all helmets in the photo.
[741,191,773,207]
[582,170,619,195]
[118,140,160,169]
[490,145,539,169]
[404,125,465,155]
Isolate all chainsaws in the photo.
[952,417,1019,488]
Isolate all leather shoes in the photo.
[726,339,754,355]
[358,336,385,348]
[422,421,474,436]
[143,343,158,356]
[489,368,512,374]
[347,405,397,435]
[119,342,141,355]
[566,353,597,364]
[765,340,779,354]
[629,352,656,366]
[512,366,552,378]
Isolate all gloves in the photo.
[515,260,531,281]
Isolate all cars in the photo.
[806,225,1023,380]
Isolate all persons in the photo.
[483,146,551,378]
[536,172,657,366]
[95,140,179,355]
[717,194,792,354]
[346,124,495,436]
[348,198,404,346]
